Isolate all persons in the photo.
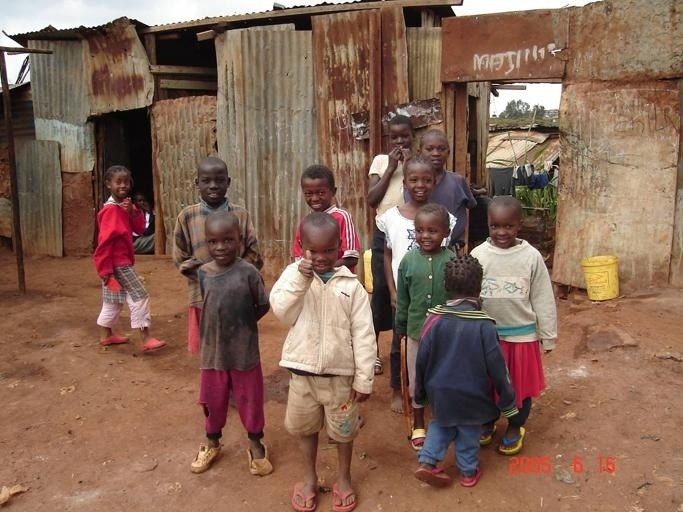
[171,157,265,410]
[402,129,477,257]
[189,210,274,476]
[130,193,155,254]
[292,164,366,445]
[393,203,457,452]
[375,155,457,414]
[92,165,168,353]
[365,116,416,375]
[413,254,520,489]
[267,210,378,512]
[466,195,559,457]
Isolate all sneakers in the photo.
[191,443,224,473]
[246,442,273,475]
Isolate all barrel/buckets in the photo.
[579,254,621,301]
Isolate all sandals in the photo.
[141,339,167,353]
[100,335,129,346]
[375,358,383,375]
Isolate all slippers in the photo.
[415,468,451,488]
[499,427,526,456]
[332,481,356,512]
[411,427,426,450]
[460,467,482,487]
[292,480,316,512]
[479,424,496,444]
